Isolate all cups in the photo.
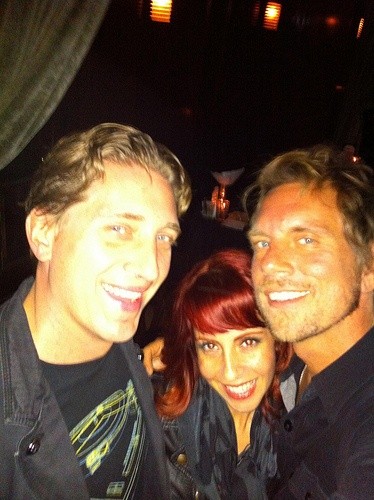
[216,199,230,218]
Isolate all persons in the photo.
[0,122,191,500]
[150,248,298,500]
[141,145,373,500]
